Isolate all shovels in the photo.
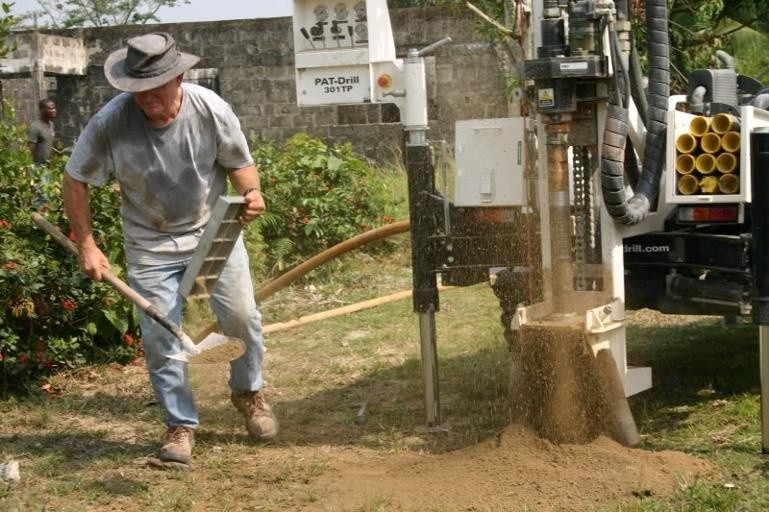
[30,211,246,365]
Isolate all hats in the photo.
[102,31,205,94]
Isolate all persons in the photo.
[28,97,57,170]
[60,32,278,463]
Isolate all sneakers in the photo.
[158,422,197,464]
[229,387,280,441]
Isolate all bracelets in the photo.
[243,187,259,197]
